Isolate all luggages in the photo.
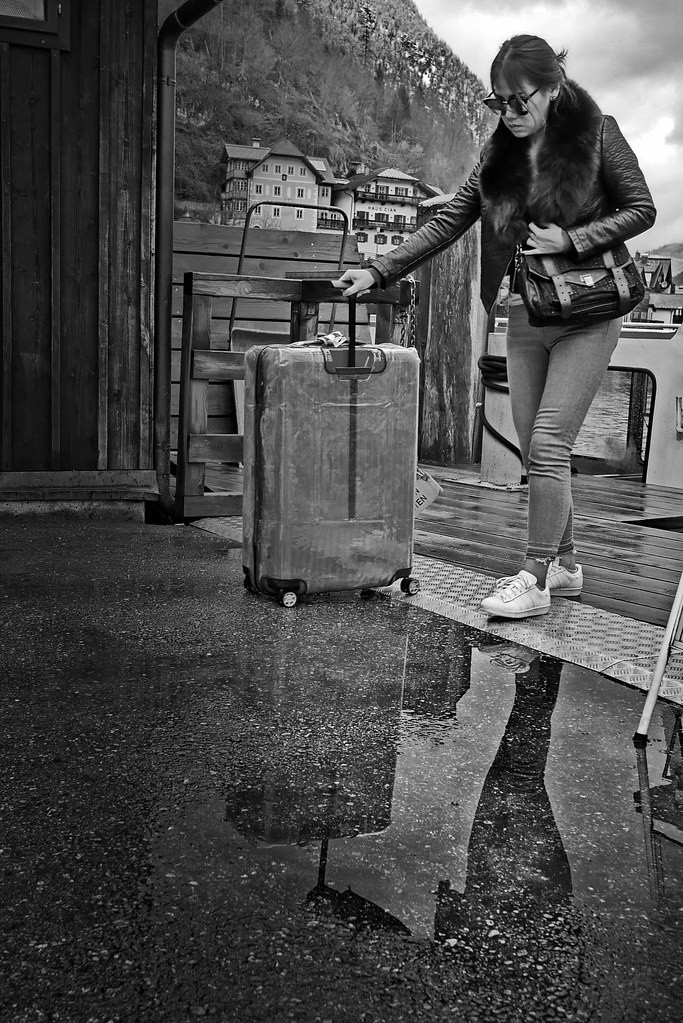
[242,283,422,606]
[222,603,408,890]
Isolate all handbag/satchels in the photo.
[515,239,646,331]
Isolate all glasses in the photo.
[483,83,543,115]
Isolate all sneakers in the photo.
[481,568,552,618]
[496,556,585,597]
[478,640,538,675]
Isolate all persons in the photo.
[339,34,656,618]
[435,638,574,948]
